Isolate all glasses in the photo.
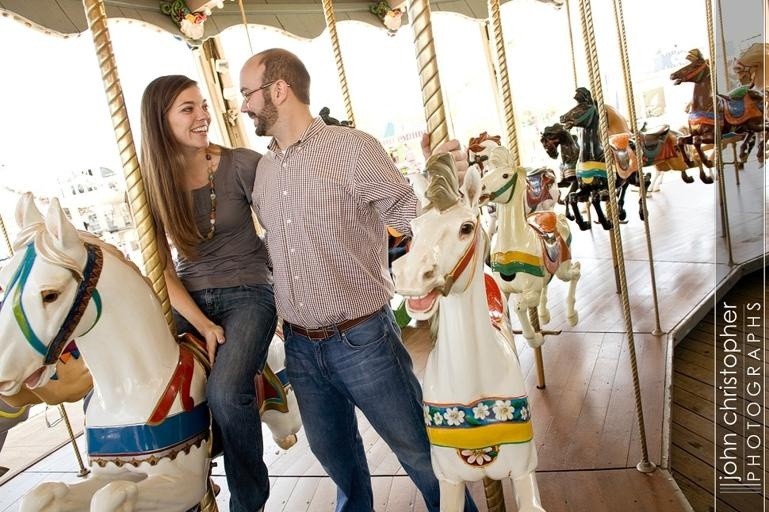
[242,81,291,103]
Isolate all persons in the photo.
[141,49,478,512]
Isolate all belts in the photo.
[283,310,380,340]
